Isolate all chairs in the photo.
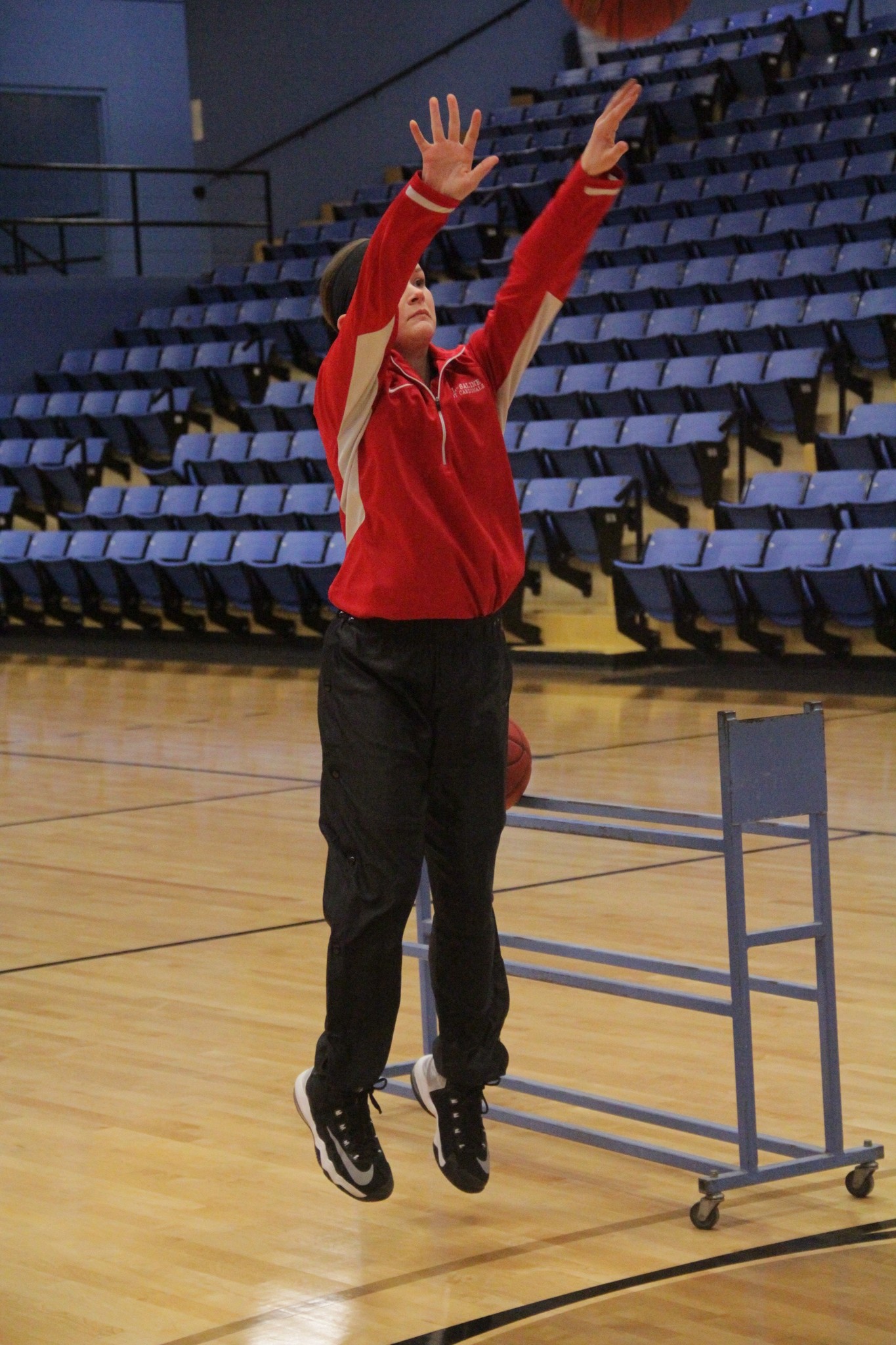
[0,0,896,657]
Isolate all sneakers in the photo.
[292,1065,397,1201]
[411,1054,501,1190]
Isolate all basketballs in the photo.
[556,0,695,47]
[504,718,534,812]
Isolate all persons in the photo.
[294,79,636,1202]
